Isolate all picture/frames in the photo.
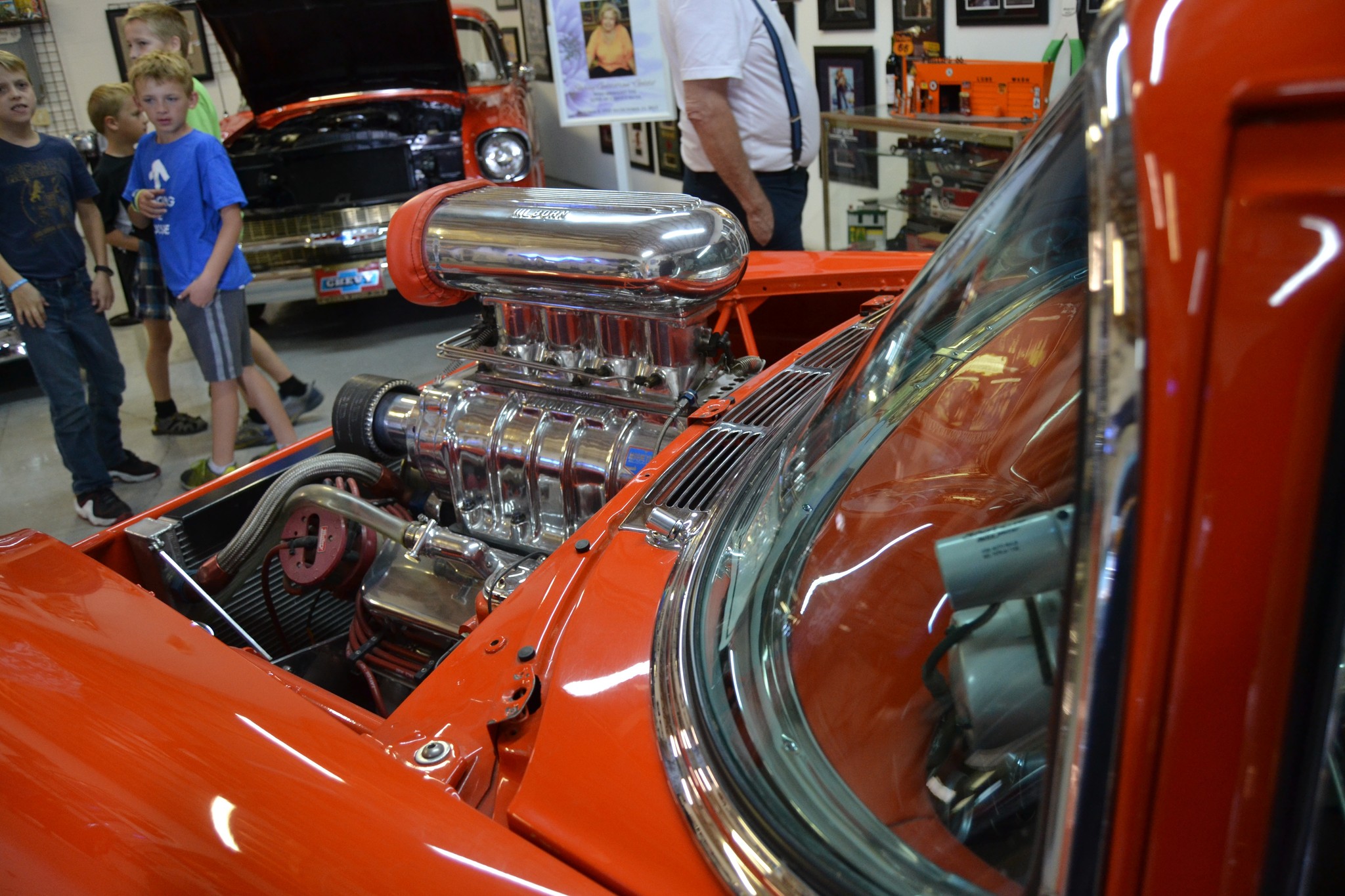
[812,45,880,190]
[956,0,1052,27]
[890,0,947,75]
[814,0,876,35]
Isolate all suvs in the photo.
[177,0,546,321]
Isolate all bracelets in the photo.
[131,189,145,213]
[94,265,114,277]
[8,278,28,293]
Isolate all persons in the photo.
[121,49,298,491]
[658,1,821,252]
[586,2,636,79]
[86,83,212,437]
[835,67,849,110]
[1,49,162,527]
[121,0,324,450]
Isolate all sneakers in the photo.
[248,444,289,463]
[150,412,211,441]
[232,416,276,449]
[106,448,161,484]
[179,458,239,491]
[73,487,132,527]
[279,378,323,426]
